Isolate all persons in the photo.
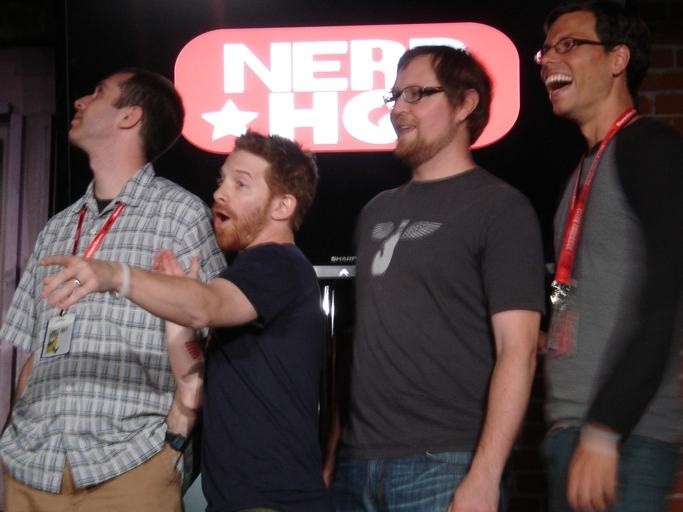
[36,129,331,510]
[322,44,549,512]
[0,70,228,510]
[531,0,682,512]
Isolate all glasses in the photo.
[383,85,446,110]
[534,38,605,65]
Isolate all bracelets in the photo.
[585,422,621,442]
[116,262,130,297]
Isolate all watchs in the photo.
[165,430,186,452]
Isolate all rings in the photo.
[72,277,80,285]
